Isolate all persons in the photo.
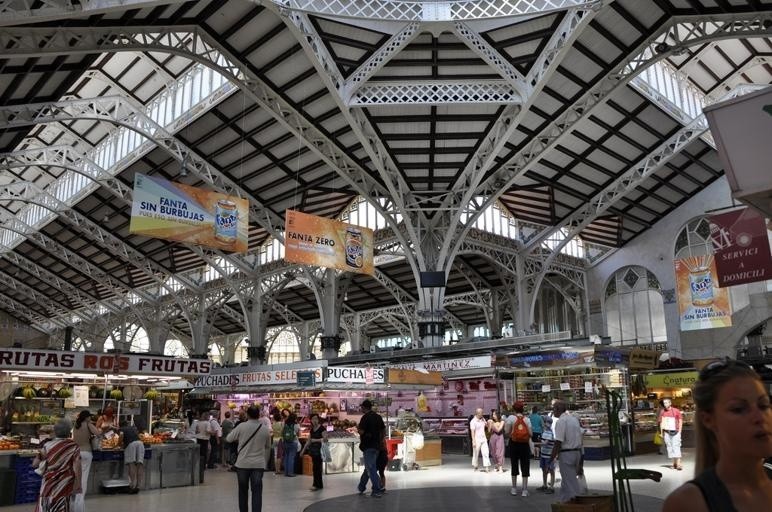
[70,410,102,512]
[184,404,302,477]
[501,400,587,503]
[300,414,329,491]
[658,398,683,470]
[662,356,772,512]
[225,405,271,512]
[32,419,82,512]
[470,407,491,472]
[358,438,388,493]
[95,407,120,433]
[116,420,145,489]
[358,399,386,498]
[448,394,466,417]
[487,409,505,472]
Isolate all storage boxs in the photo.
[10,455,43,504]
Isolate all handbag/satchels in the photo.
[91,434,103,450]
[320,446,331,462]
[35,459,47,475]
[229,452,238,465]
[661,415,675,431]
[69,492,86,511]
[654,432,664,446]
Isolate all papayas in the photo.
[331,419,351,431]
[311,401,326,414]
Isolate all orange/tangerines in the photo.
[142,435,162,444]
[0,439,21,450]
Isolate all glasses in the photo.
[707,356,751,371]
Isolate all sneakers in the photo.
[536,486,547,490]
[511,487,517,495]
[522,490,531,496]
[545,487,555,494]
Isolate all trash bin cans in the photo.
[0,468,16,506]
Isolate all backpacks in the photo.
[282,422,296,443]
[511,414,529,443]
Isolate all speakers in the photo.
[420,271,445,288]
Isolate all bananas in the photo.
[57,385,74,398]
[21,386,38,398]
[109,385,122,399]
[142,390,159,400]
[417,392,429,412]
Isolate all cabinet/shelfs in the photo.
[422,418,469,436]
[11,396,116,425]
[515,365,631,438]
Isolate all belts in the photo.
[559,448,579,452]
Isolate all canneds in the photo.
[343,227,364,268]
[215,199,239,247]
[690,266,715,308]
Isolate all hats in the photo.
[513,401,524,410]
[79,410,94,416]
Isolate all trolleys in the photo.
[549,388,664,512]
[389,423,421,472]
